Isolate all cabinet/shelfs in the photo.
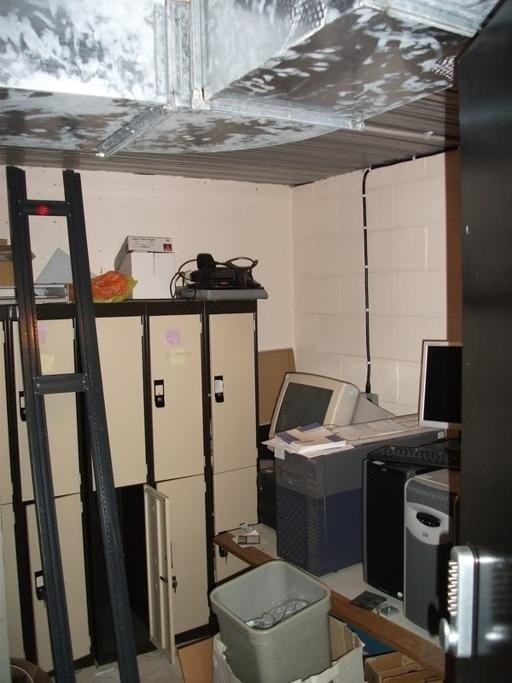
[1,298,262,681]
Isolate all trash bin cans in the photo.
[210,559,332,683]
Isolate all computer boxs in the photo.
[275,414,448,576]
[404,468,463,636]
[363,458,436,602]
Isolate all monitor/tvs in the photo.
[267,373,396,454]
[418,340,462,452]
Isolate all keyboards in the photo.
[367,444,461,470]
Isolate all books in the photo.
[274,422,348,455]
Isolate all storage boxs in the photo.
[114,235,177,300]
[174,559,443,683]
